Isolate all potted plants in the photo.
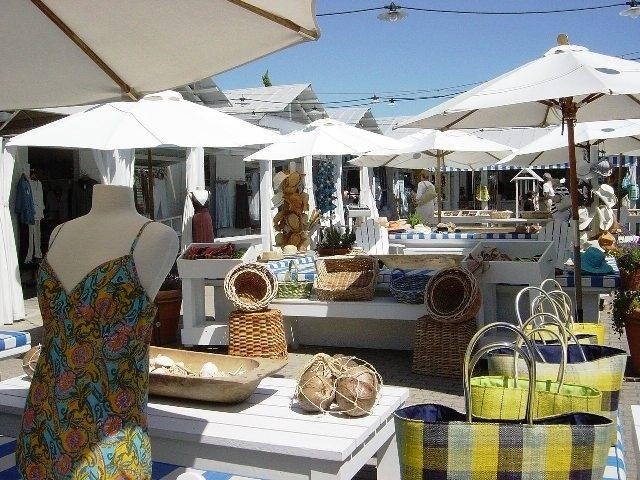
[317,227,356,255]
[609,291,640,376]
[607,244,640,290]
[151,276,181,346]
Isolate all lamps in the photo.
[378,3,408,24]
[619,2,640,19]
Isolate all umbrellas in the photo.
[495,119,640,205]
[5,88,295,219]
[347,131,517,223]
[391,34,640,324]
[0,0,322,113]
[243,118,422,230]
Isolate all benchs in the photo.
[270,296,430,349]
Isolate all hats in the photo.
[578,181,594,207]
[301,193,309,211]
[285,213,303,233]
[571,247,613,274]
[570,231,590,249]
[578,206,594,230]
[285,193,304,215]
[598,230,615,251]
[591,159,613,176]
[273,210,286,231]
[297,234,310,252]
[299,213,309,228]
[596,205,613,231]
[286,171,306,192]
[285,232,306,247]
[594,184,617,208]
[273,233,286,247]
[273,171,289,190]
[271,192,285,210]
[576,160,594,179]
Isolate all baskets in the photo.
[412,314,481,379]
[230,268,271,306]
[390,267,432,304]
[223,261,279,311]
[425,264,482,323]
[430,274,469,316]
[313,258,376,301]
[228,309,289,364]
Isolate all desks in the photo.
[0,376,410,479]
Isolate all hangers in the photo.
[238,178,247,186]
[29,169,39,181]
[18,168,28,181]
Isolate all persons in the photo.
[543,173,555,212]
[411,170,435,224]
[189,186,214,243]
[14,184,179,480]
[547,179,571,221]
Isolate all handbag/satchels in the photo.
[512,290,598,345]
[393,322,614,480]
[515,278,605,345]
[555,191,572,212]
[486,286,628,447]
[469,313,603,422]
[419,181,437,205]
